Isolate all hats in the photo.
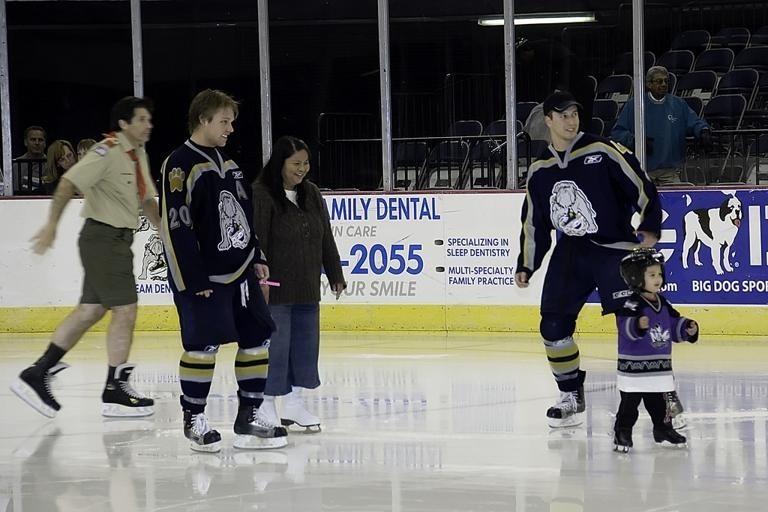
[543,91,583,116]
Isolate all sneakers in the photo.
[667,390,684,419]
[19,355,71,410]
[102,363,155,408]
[233,390,289,438]
[261,394,282,428]
[178,395,222,445]
[653,422,686,444]
[546,383,585,418]
[613,425,634,448]
[278,391,320,428]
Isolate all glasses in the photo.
[650,77,669,85]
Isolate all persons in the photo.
[11,97,160,418]
[160,91,289,454]
[607,66,712,186]
[251,137,346,433]
[11,127,49,190]
[47,140,76,186]
[78,139,96,161]
[514,91,686,430]
[612,246,699,453]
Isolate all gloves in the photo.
[631,135,655,156]
[700,129,715,146]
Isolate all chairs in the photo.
[375,142,431,191]
[668,71,680,95]
[675,30,711,57]
[483,120,523,139]
[592,116,604,136]
[594,98,619,135]
[503,101,540,124]
[692,48,735,83]
[685,92,747,183]
[596,74,634,115]
[681,97,704,120]
[682,71,718,106]
[611,51,656,75]
[735,46,768,86]
[714,67,758,111]
[448,119,483,146]
[418,140,469,193]
[665,49,695,74]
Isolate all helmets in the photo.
[618,247,667,293]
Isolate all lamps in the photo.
[477,11,598,27]
